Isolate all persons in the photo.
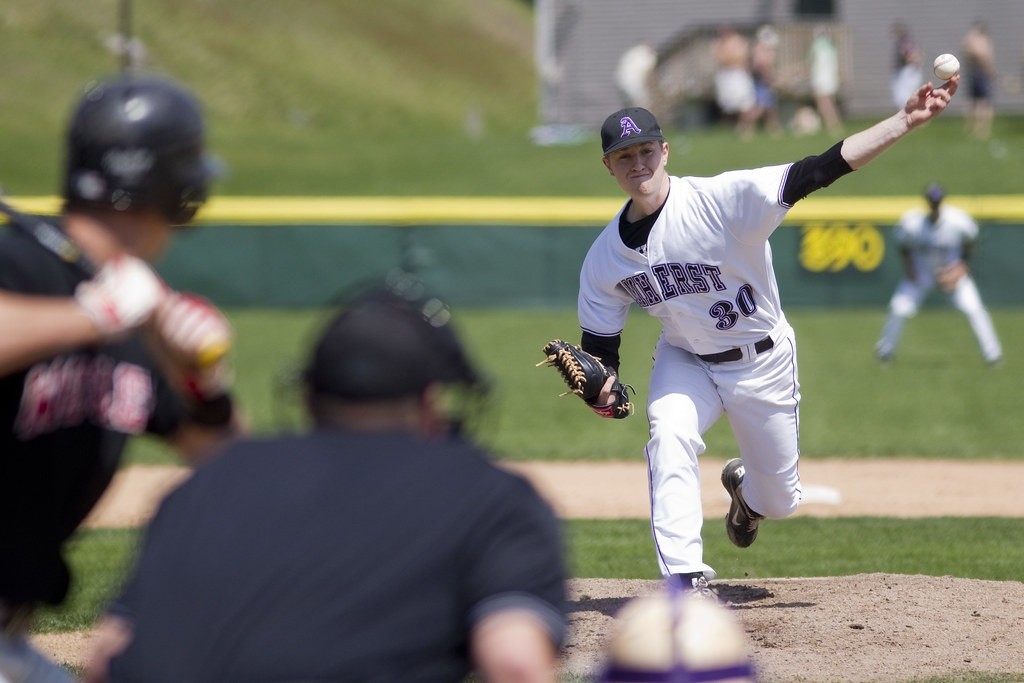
[85,282,570,683]
[873,183,1003,364]
[614,16,997,141]
[0,73,241,683]
[576,76,961,600]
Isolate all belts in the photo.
[698,337,774,364]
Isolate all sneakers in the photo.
[720,457,767,549]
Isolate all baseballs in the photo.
[933,53,960,80]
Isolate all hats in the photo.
[600,107,665,156]
[924,182,943,203]
[302,286,490,404]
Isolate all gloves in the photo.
[153,290,235,397]
[73,253,173,340]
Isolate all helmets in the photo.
[61,67,222,209]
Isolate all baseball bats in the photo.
[0,201,232,366]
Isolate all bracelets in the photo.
[172,388,231,431]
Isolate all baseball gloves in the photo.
[937,263,965,293]
[542,339,630,419]
